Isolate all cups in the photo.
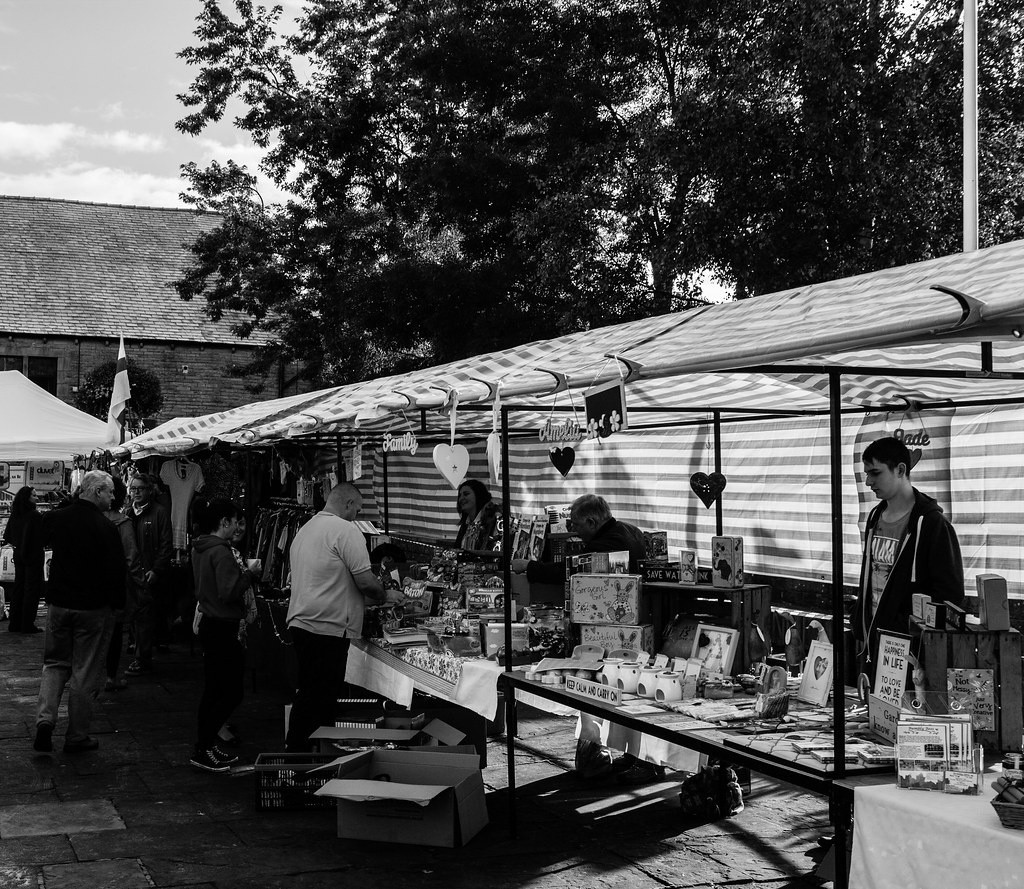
[247,558,261,569]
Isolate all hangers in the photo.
[258,497,313,513]
[172,448,276,470]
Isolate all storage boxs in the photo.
[679,550,698,586]
[713,536,745,587]
[254,678,506,847]
[912,594,967,632]
[570,573,656,659]
[976,574,1011,631]
[547,533,589,571]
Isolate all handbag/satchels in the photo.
[192,600,203,635]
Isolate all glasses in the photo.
[130,486,149,492]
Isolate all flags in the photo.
[105,331,132,447]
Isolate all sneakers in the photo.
[190,749,231,772]
[208,745,239,764]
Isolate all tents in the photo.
[0,236,1024,889]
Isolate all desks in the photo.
[909,614,1024,754]
[345,636,520,736]
[642,584,771,676]
[499,672,896,838]
[830,750,1024,889]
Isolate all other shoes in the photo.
[124,661,151,675]
[104,681,120,691]
[575,750,611,778]
[8,622,44,635]
[617,759,665,784]
[612,752,638,770]
[33,723,54,752]
[63,735,99,752]
[817,835,835,851]
[126,641,135,653]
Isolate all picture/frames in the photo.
[584,378,629,439]
[691,624,740,675]
[797,640,833,708]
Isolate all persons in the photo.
[124,469,172,675]
[193,510,256,744]
[285,482,385,752]
[453,480,503,553]
[4,486,57,633]
[54,487,135,685]
[571,494,651,573]
[187,497,264,771]
[33,471,123,755]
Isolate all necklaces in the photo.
[855,437,964,687]
